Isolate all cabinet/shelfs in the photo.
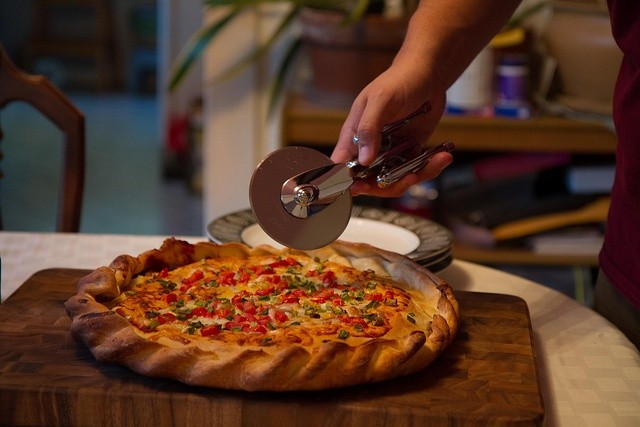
[279,85,615,266]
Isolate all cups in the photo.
[495,53,531,108]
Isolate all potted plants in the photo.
[162,0,418,125]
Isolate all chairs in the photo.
[1,48,87,233]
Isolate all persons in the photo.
[329,0,639,350]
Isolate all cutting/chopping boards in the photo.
[0,266,547,427]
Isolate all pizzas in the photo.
[65,240,460,393]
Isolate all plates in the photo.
[205,204,457,263]
[416,247,454,268]
[428,255,454,275]
